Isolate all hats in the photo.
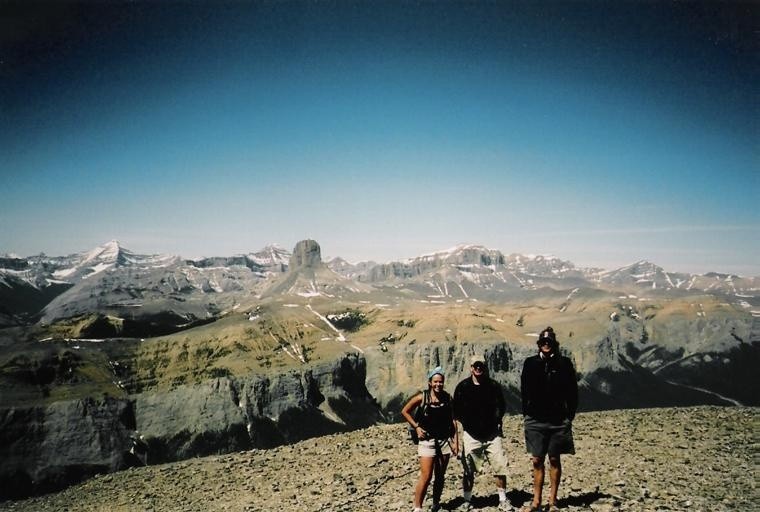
[470,354,486,366]
[537,327,556,344]
[428,369,445,379]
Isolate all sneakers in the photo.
[459,502,473,512]
[545,503,559,512]
[498,498,513,512]
[432,507,449,512]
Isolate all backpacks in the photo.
[407,390,453,456]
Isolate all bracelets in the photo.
[415,425,420,430]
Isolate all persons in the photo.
[518,327,579,511]
[449,353,513,512]
[400,368,461,511]
